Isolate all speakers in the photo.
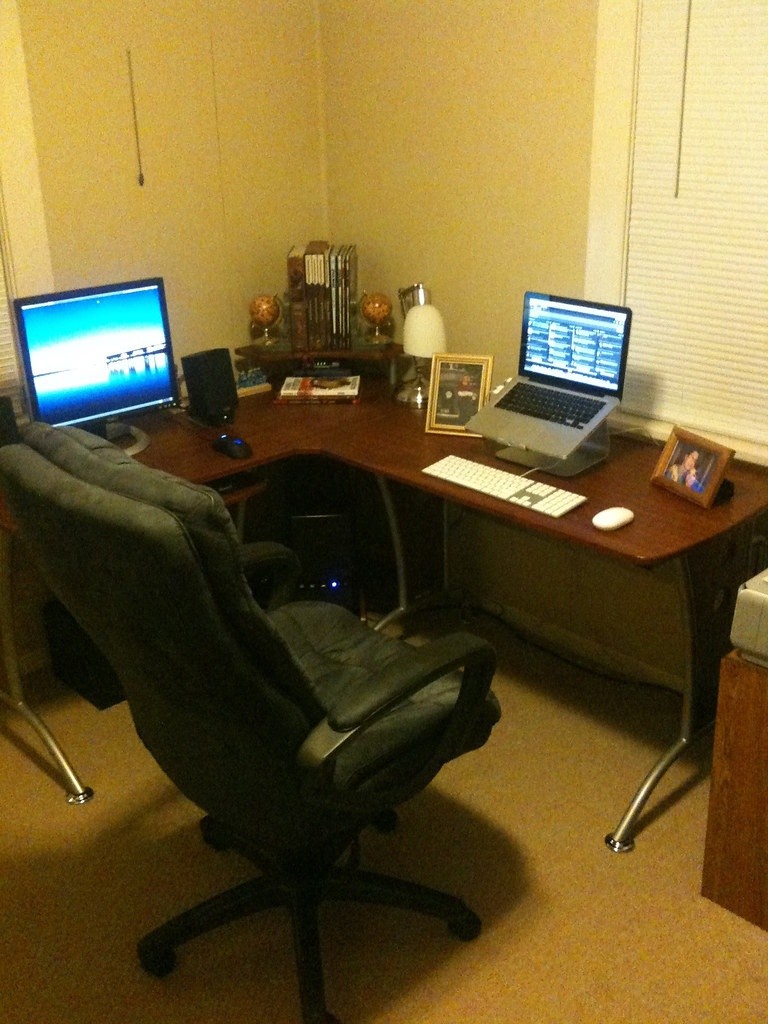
[181,348,239,427]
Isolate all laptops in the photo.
[466,292,632,459]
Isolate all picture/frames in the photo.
[650,425,736,511]
[425,353,494,438]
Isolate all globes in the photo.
[357,290,394,346]
[250,292,286,347]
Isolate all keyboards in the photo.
[421,455,587,518]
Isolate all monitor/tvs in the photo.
[13,277,179,456]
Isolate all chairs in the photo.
[0,420,502,1023]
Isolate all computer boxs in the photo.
[290,487,359,617]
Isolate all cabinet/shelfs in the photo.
[701,648,768,932]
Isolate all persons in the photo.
[457,373,477,425]
[665,447,704,495]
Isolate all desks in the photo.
[1,373,768,853]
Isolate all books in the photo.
[286,239,359,354]
[272,376,364,405]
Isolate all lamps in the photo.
[392,282,445,410]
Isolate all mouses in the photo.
[591,507,634,531]
[213,434,251,459]
